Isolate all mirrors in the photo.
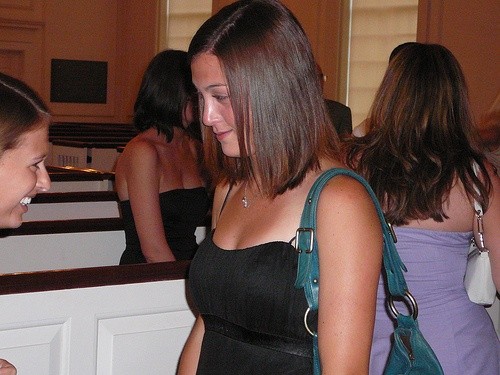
[0,0,500,295]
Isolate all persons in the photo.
[0,72,54,375]
[176,0,384,375]
[114,48,212,267]
[341,41,500,375]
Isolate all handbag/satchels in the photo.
[294,167,445,375]
[463,161,496,305]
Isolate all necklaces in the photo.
[242,180,259,207]
